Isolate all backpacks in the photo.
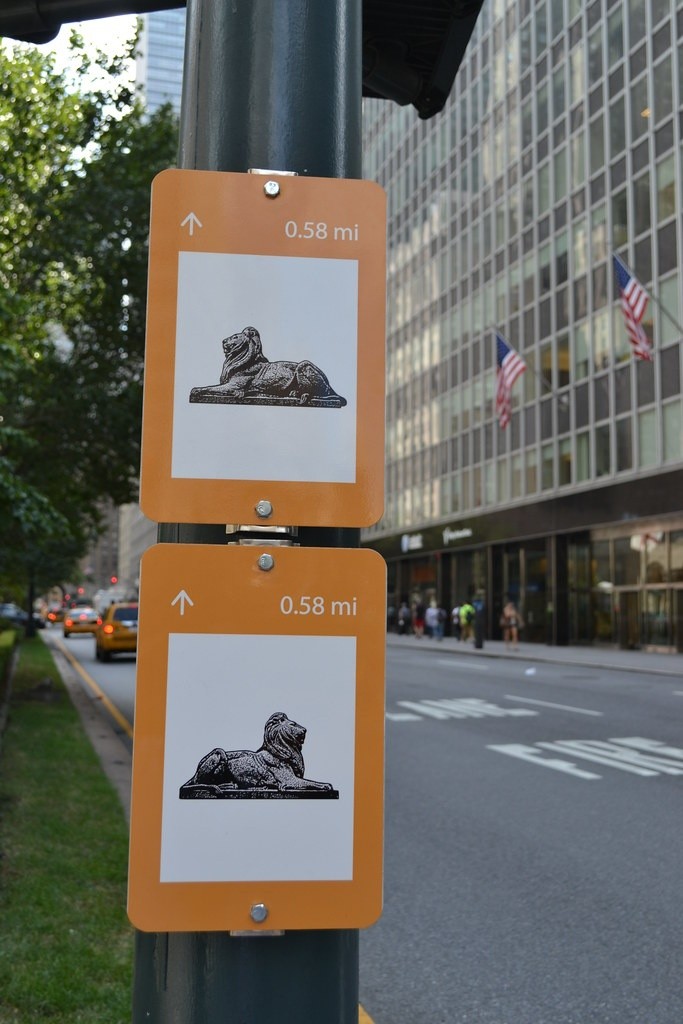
[464,605,472,623]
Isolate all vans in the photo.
[95,593,122,613]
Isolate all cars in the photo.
[64,608,103,638]
[45,608,65,624]
[1,605,47,629]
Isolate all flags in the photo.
[615,259,652,361]
[495,336,527,431]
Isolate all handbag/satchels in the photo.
[438,609,443,624]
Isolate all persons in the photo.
[388,598,520,648]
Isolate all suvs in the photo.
[96,601,139,663]
